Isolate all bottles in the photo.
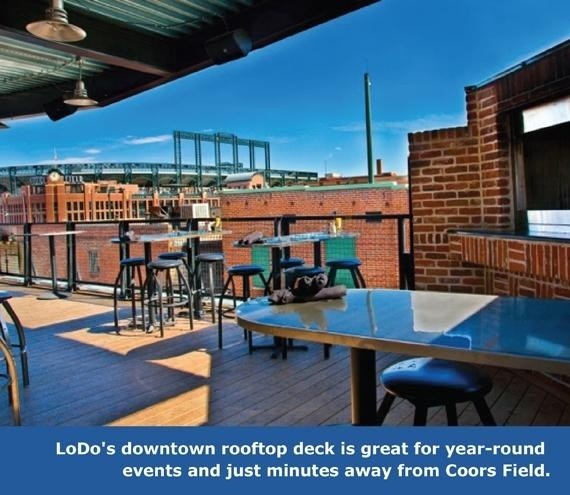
[327,215,344,237]
[205,217,224,234]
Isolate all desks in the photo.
[15,228,90,300]
[110,228,234,331]
[234,284,568,426]
[232,230,362,362]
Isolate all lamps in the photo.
[25,0,88,42]
[63,54,98,107]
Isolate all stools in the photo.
[368,355,497,427]
[112,252,367,362]
